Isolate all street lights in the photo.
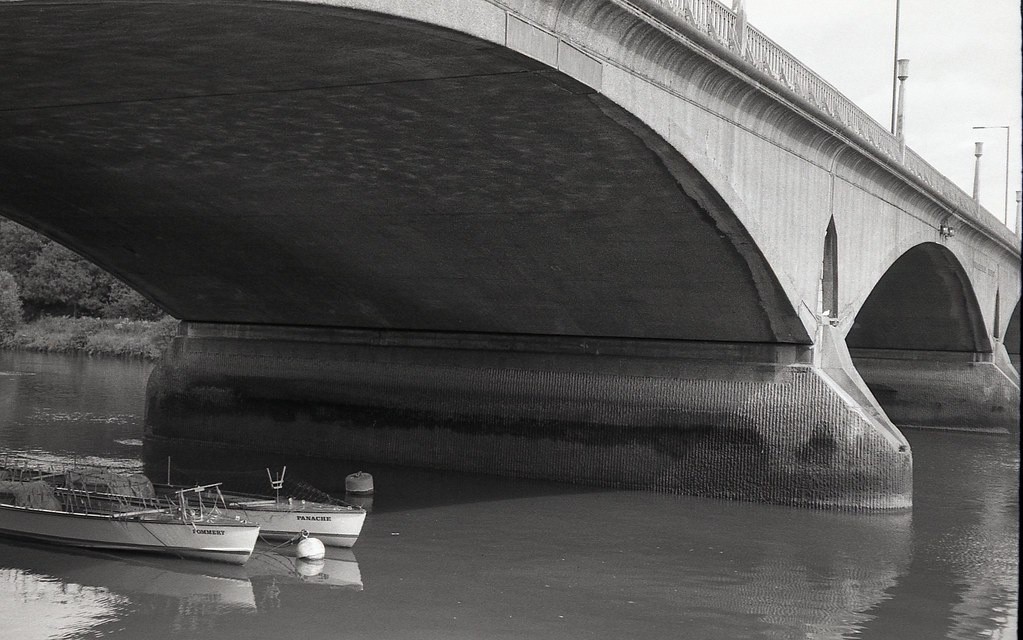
[973,125,1009,227]
[974,141,983,203]
[896,58,911,136]
[1015,191,1022,237]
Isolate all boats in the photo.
[1,481,261,565]
[242,539,366,593]
[0,535,257,610]
[154,466,367,549]
